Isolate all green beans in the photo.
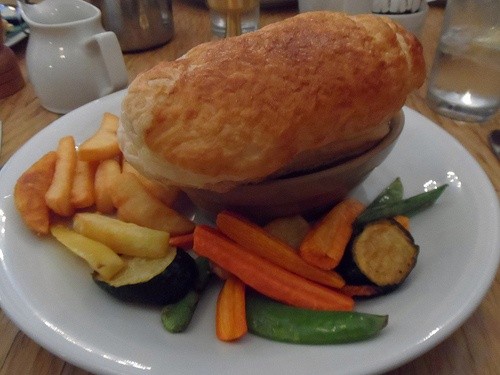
[350,178,449,233]
[246,296,390,343]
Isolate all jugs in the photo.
[17,0,128,114]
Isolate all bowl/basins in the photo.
[180,109,405,225]
[373,3,429,36]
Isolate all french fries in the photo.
[14,112,213,278]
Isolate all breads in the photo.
[117,11,426,189]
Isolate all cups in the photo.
[208,0,259,38]
[91,0,173,52]
[426,0,500,119]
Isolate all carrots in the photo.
[168,198,366,342]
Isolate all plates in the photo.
[3,29,29,47]
[0,86,500,375]
